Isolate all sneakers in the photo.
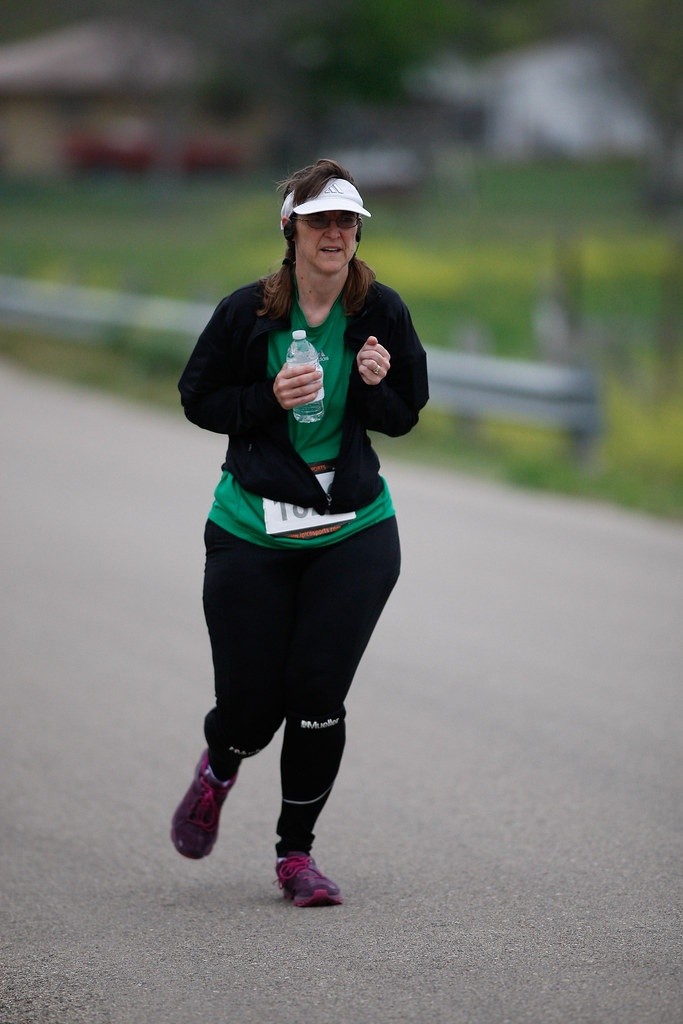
[274,851,346,906]
[171,748,238,860]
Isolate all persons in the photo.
[171,158,429,907]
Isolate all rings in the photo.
[372,365,381,374]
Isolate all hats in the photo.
[278,178,372,231]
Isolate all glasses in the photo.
[294,212,361,230]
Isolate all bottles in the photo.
[286,329,324,424]
